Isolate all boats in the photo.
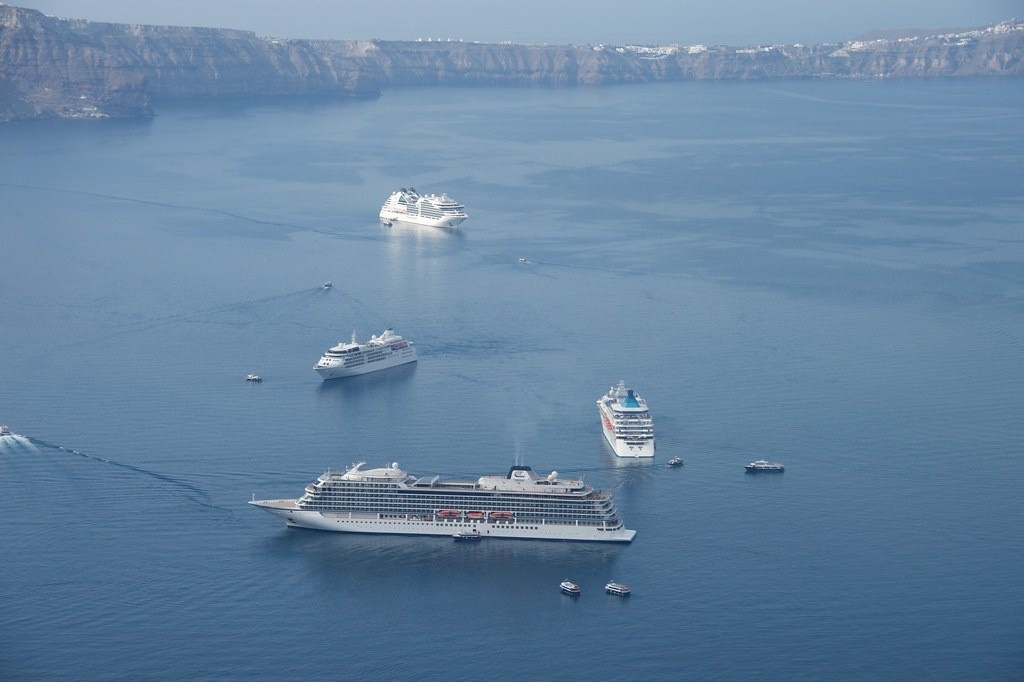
[467,513,483,519]
[559,581,580,595]
[596,380,657,459]
[489,512,513,519]
[437,510,460,518]
[605,582,631,596]
[743,459,785,473]
[450,531,482,541]
[666,455,684,469]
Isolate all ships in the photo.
[246,462,638,543]
[378,186,469,228]
[312,327,420,380]
